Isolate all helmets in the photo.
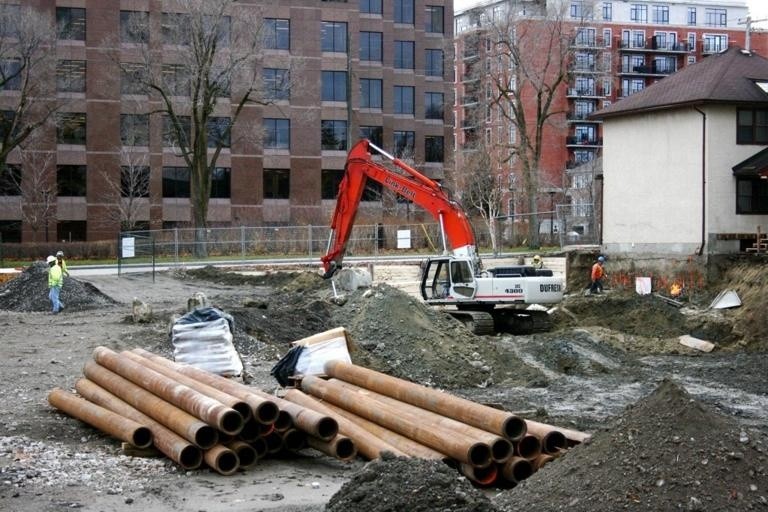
[598,257,604,262]
[534,255,540,260]
[46,255,57,264]
[56,251,64,257]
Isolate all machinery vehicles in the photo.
[316,138,563,336]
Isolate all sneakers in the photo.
[48,306,65,315]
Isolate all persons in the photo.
[377,223,384,253]
[47,255,64,315]
[531,255,543,269]
[56,251,69,277]
[592,256,608,293]
